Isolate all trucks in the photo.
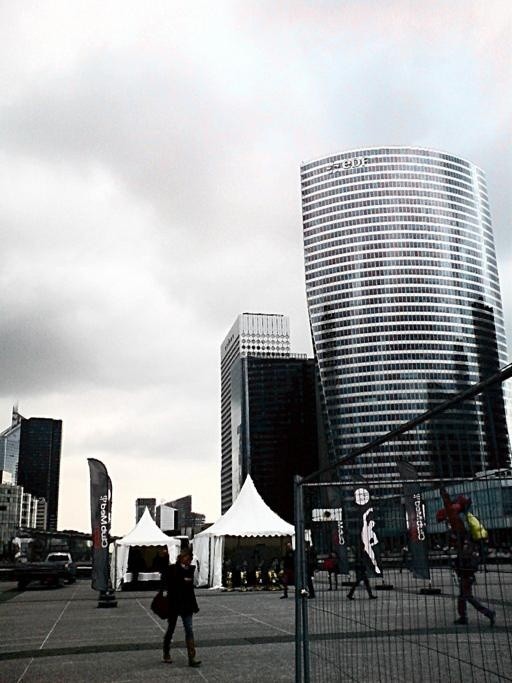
[13,552,77,589]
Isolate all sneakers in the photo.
[279,594,289,600]
[308,591,316,600]
[488,609,496,627]
[454,615,468,625]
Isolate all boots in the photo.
[368,592,377,600]
[161,637,172,664]
[186,636,201,668]
[346,592,355,600]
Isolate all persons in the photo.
[279,540,298,599]
[327,550,341,590]
[397,544,412,573]
[345,542,377,601]
[158,547,203,667]
[221,553,286,593]
[434,492,496,628]
[359,506,382,575]
[303,539,317,599]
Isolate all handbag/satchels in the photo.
[150,590,173,622]
[279,570,296,586]
